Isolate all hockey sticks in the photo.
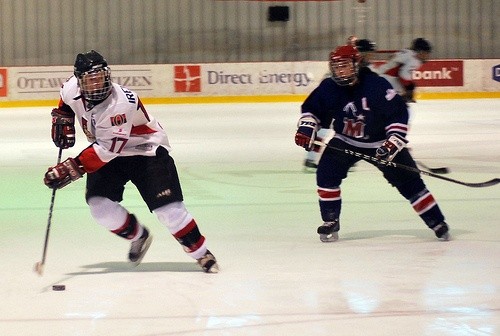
[34,135,65,275]
[314,140,500,188]
[413,160,448,174]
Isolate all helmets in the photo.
[74,50,113,105]
[329,46,363,86]
[412,37,431,52]
[356,38,375,52]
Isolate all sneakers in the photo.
[198,249,219,273]
[433,222,449,240]
[317,220,340,242]
[129,228,153,266]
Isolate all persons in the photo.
[294,36,450,242]
[45,50,219,274]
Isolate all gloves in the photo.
[295,118,317,151]
[44,158,83,190]
[375,133,409,162]
[51,108,75,149]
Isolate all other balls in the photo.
[53,285,65,290]
